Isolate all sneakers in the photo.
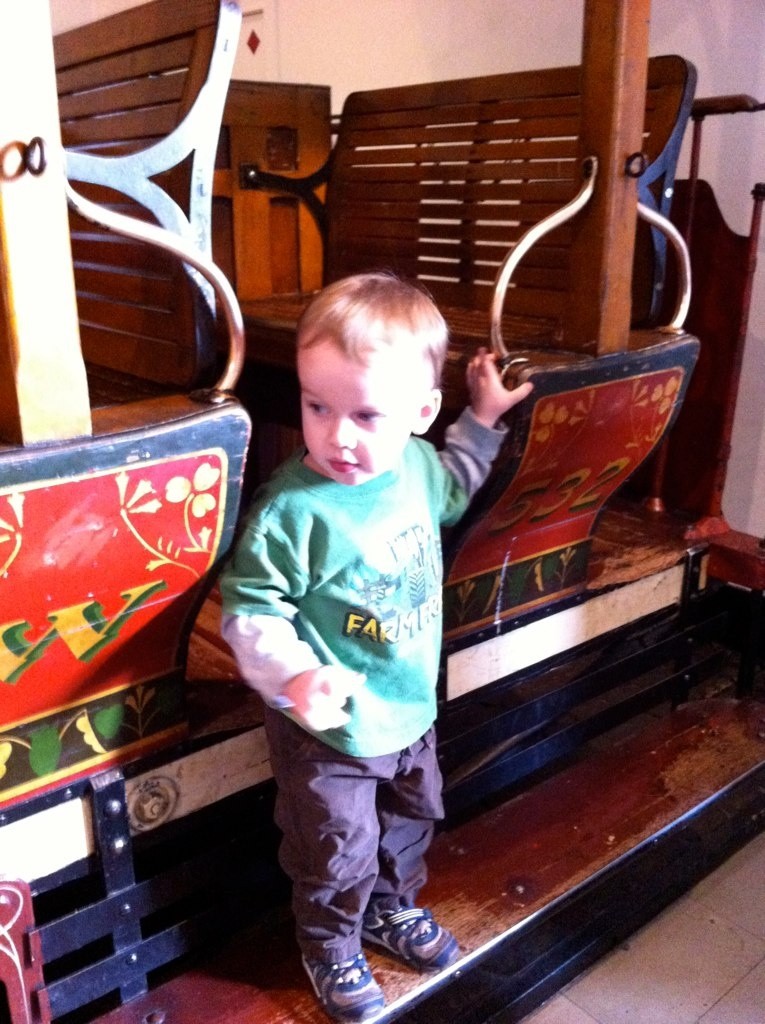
[302,951,384,1024]
[359,905,459,971]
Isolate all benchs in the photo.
[18,0,700,635]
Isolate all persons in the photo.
[219,272,532,1023]
